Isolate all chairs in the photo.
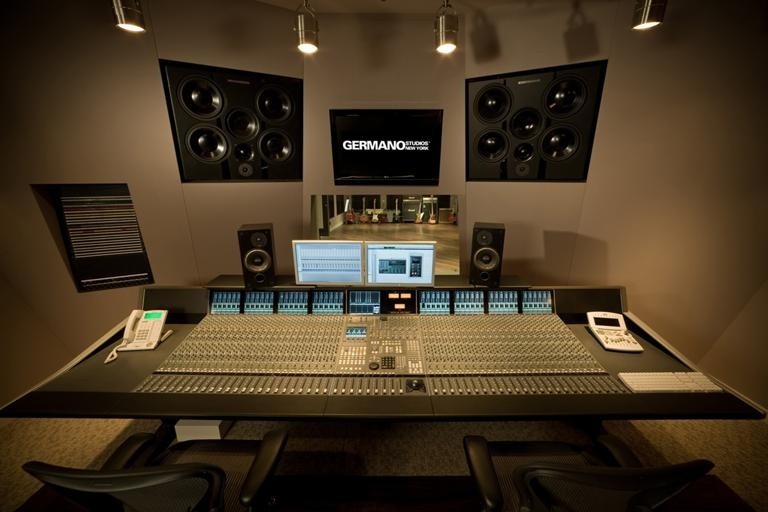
[19,431,289,511]
[464,433,714,512]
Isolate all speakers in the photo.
[401,202,456,223]
[470,222,507,288]
[463,58,609,185]
[235,223,279,287]
[159,58,305,183]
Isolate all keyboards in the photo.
[617,370,724,394]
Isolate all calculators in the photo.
[586,311,644,352]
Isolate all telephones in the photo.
[116,310,176,352]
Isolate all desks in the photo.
[0,287,764,421]
[204,275,532,292]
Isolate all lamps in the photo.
[632,0,667,30]
[434,0,458,55]
[113,0,145,33]
[294,0,318,54]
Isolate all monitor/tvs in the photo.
[293,240,364,286]
[328,106,444,186]
[364,241,437,288]
